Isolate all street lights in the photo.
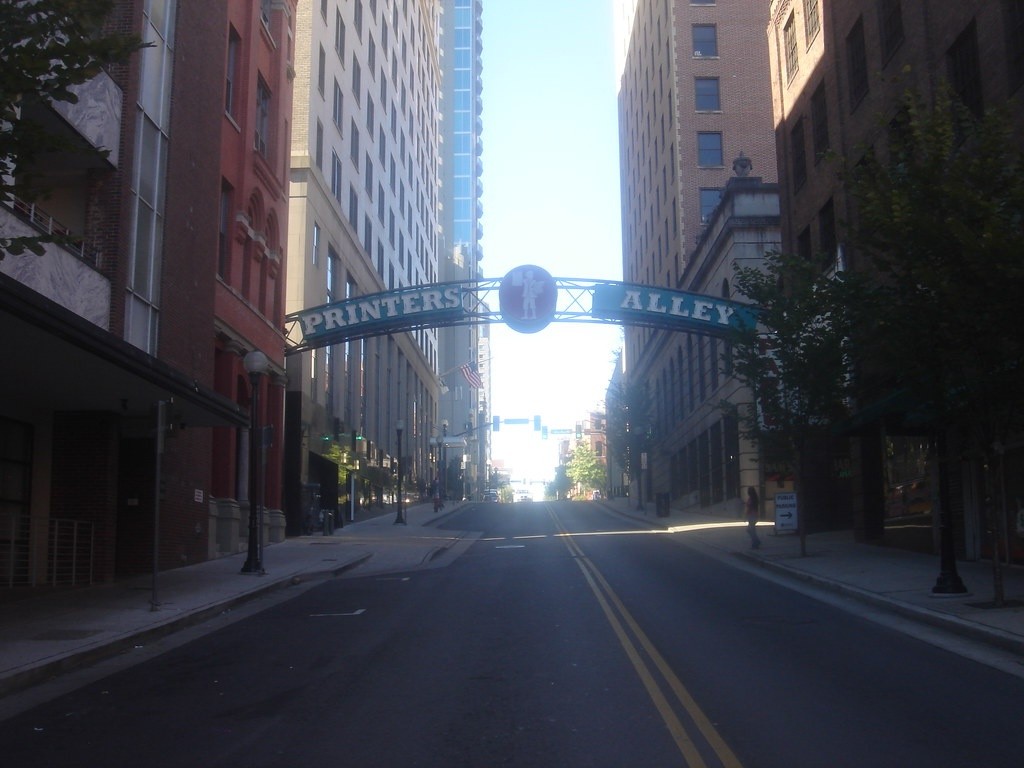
[240,351,268,573]
[395,418,405,522]
[436,435,445,507]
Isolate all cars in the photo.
[512,489,533,502]
[480,489,501,503]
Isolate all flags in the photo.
[460,360,482,388]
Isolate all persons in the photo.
[427,477,444,514]
[744,487,760,546]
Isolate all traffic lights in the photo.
[542,426,547,439]
[576,425,582,439]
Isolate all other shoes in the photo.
[751,541,761,549]
